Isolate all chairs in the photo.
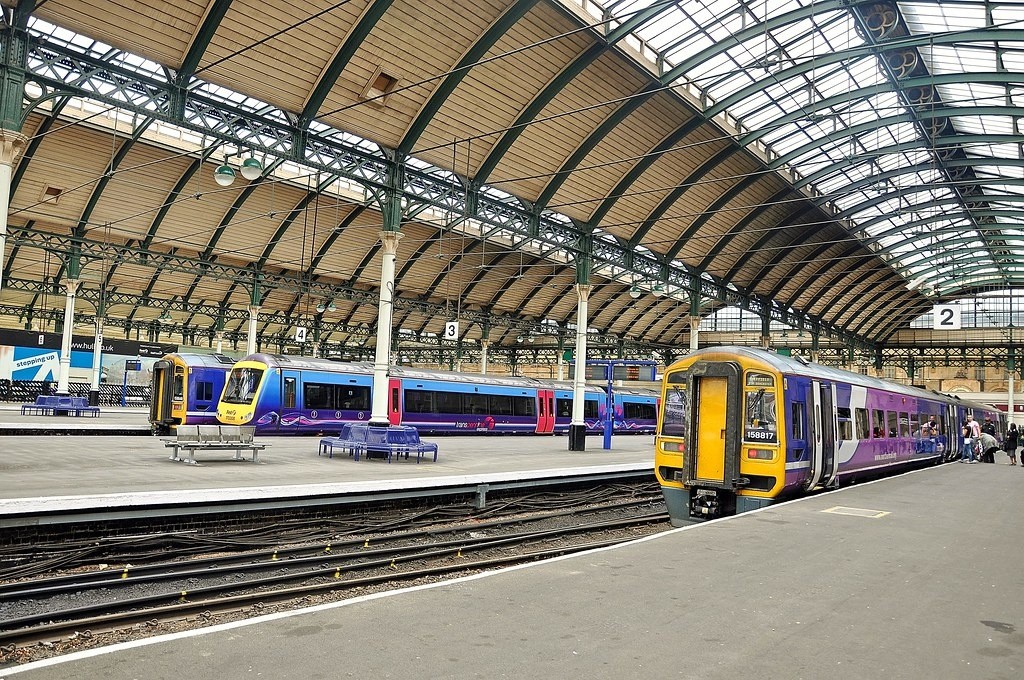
[319,423,439,464]
[21,395,101,418]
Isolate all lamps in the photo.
[630,281,641,298]
[652,279,664,297]
[157,312,164,322]
[165,312,173,321]
[328,301,336,311]
[240,149,263,180]
[517,333,523,342]
[214,156,236,186]
[316,299,325,312]
[904,274,928,291]
[528,332,535,343]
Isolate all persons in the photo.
[961,414,999,464]
[867,416,942,455]
[1004,423,1024,467]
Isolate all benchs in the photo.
[159,424,271,464]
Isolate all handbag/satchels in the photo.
[964,438,971,445]
[1002,438,1009,452]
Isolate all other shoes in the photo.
[1011,462,1016,466]
[958,459,963,463]
[967,460,973,464]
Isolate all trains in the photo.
[654,345,1009,530]
[214,352,685,437]
[147,350,240,436]
